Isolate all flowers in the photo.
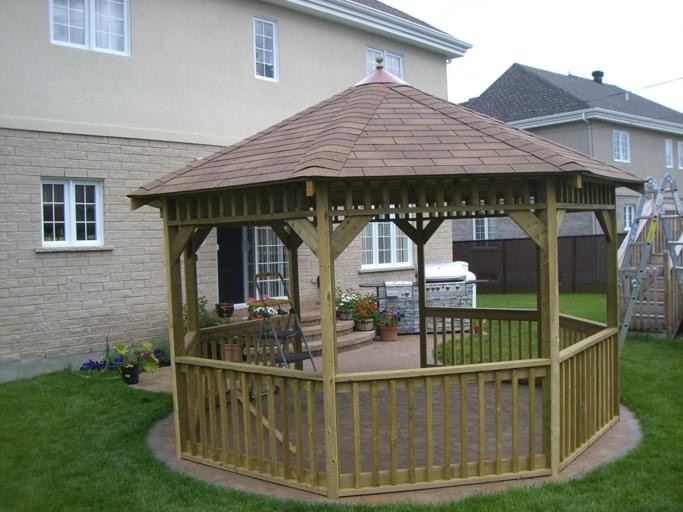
[353,297,378,318]
[375,310,405,327]
[335,286,361,310]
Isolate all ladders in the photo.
[249,272,317,401]
[617,173,683,357]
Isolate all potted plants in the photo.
[113,339,159,385]
[224,338,241,363]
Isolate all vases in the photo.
[215,303,234,318]
[354,317,374,331]
[339,310,353,320]
[377,325,399,341]
